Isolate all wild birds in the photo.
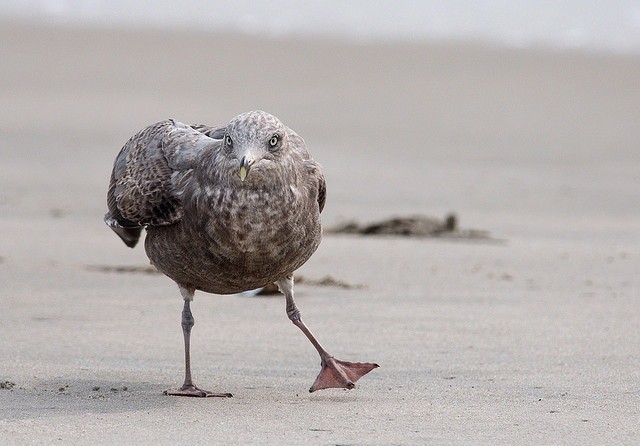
[102,109,381,399]
